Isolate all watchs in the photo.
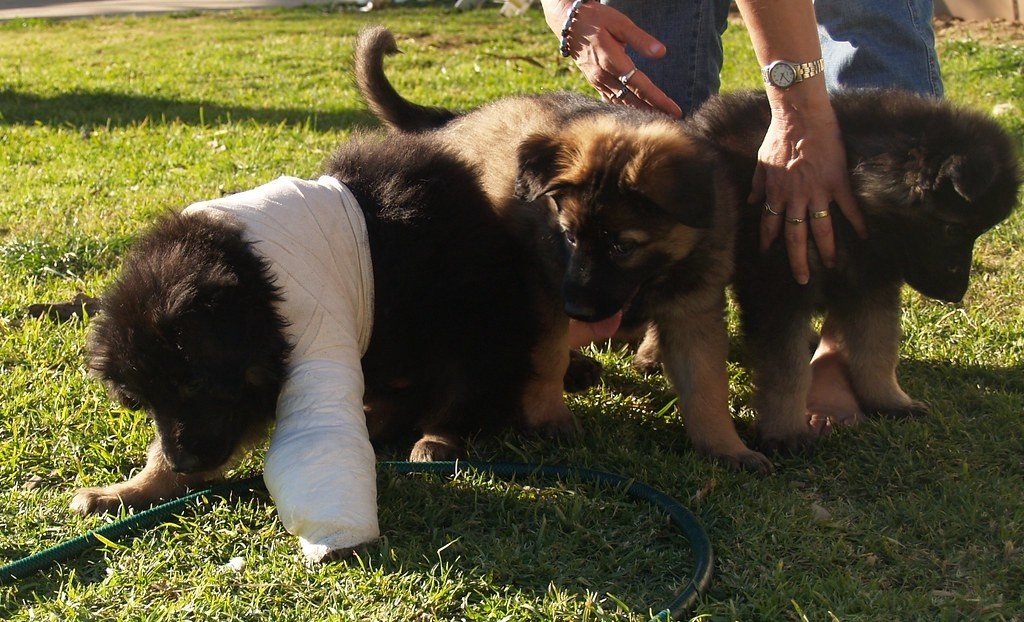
[761,59,824,89]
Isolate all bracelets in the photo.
[558,0,585,58]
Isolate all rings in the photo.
[608,93,615,100]
[785,215,806,223]
[763,202,783,215]
[808,211,829,218]
[616,87,627,98]
[618,66,637,85]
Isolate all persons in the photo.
[539,0,945,432]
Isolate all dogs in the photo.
[69,25,1024,566]
